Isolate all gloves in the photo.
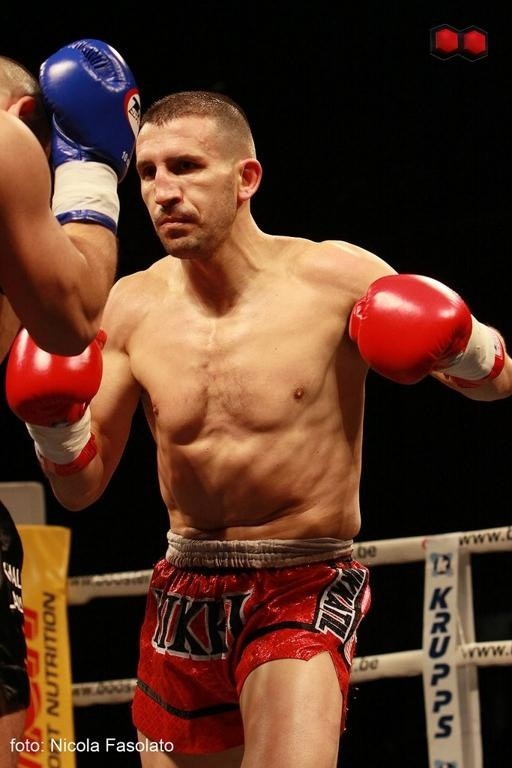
[349,275,505,387]
[6,326,107,477]
[39,39,141,234]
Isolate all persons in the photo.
[0,35,143,766]
[3,89,512,768]
